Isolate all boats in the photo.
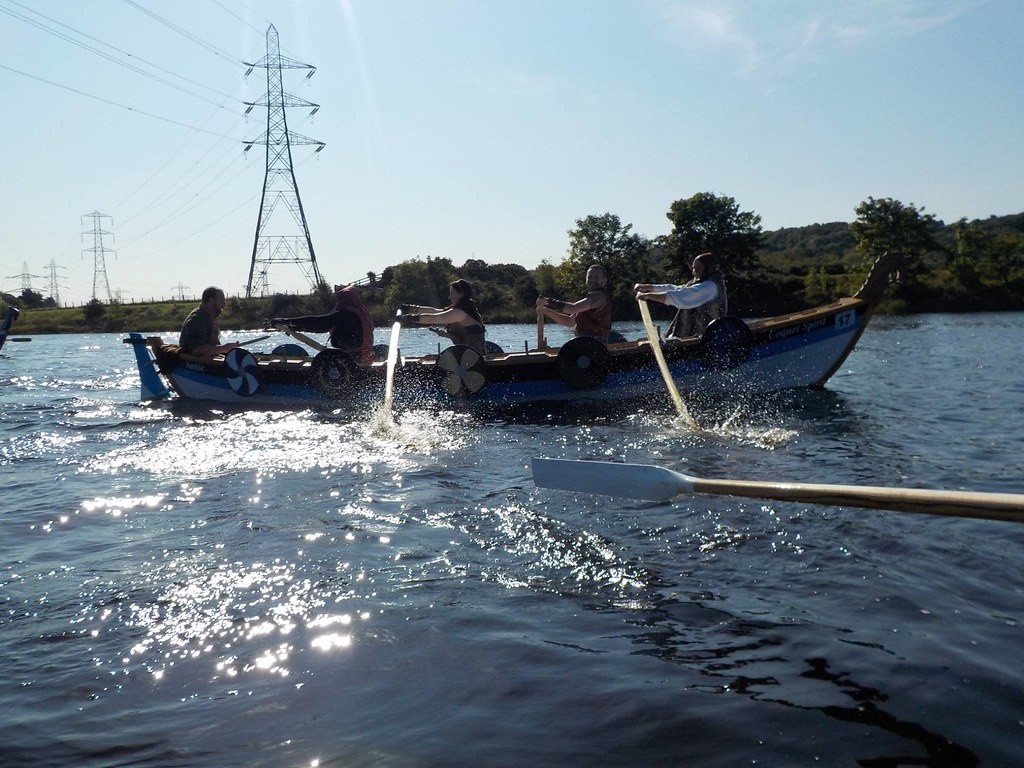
[121,250,892,417]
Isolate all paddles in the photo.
[636,289,700,430]
[6,337,32,342]
[529,456,1024,527]
[274,322,328,352]
[382,307,404,431]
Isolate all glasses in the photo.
[212,299,225,303]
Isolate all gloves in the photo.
[270,317,288,328]
[393,303,419,314]
[389,313,421,328]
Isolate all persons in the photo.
[634,254,728,339]
[179,287,238,358]
[395,280,487,357]
[535,266,612,345]
[271,286,375,363]
